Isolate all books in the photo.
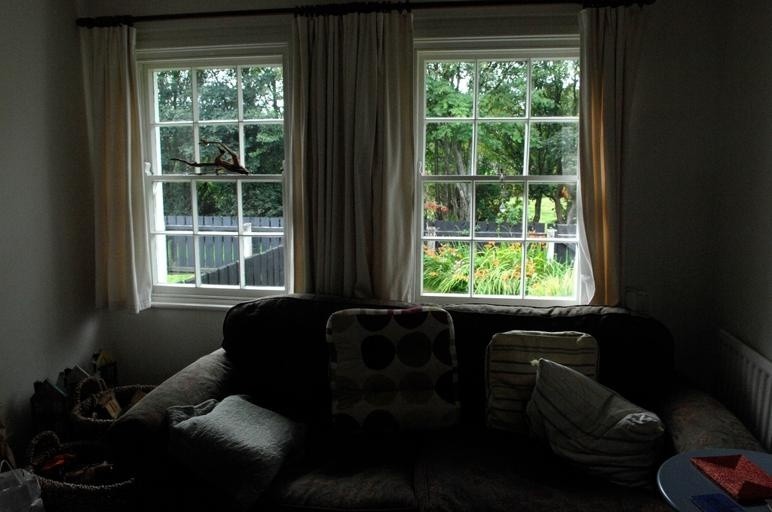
[688,493,746,512]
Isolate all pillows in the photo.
[485,329,599,434]
[326,307,462,426]
[526,357,664,488]
[166,392,307,512]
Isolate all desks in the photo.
[656,448,772,512]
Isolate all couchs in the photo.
[115,293,766,512]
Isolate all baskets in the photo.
[29,430,137,511]
[74,376,158,426]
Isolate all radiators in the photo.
[719,328,772,456]
